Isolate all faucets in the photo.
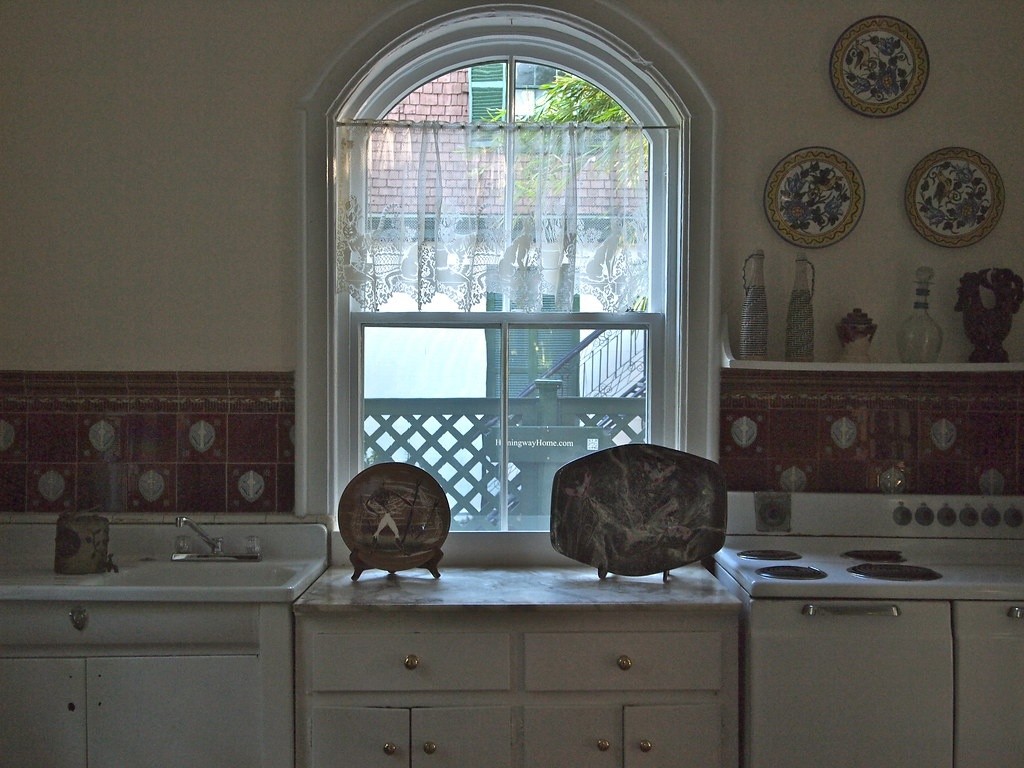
[175,515,224,555]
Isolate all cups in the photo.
[55,511,109,576]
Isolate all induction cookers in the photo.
[722,490,1024,601]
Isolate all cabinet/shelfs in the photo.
[712,547,1024,768]
[0,560,328,764]
[293,562,738,767]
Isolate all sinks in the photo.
[89,560,308,602]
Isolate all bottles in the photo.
[785,253,817,362]
[897,268,943,363]
[739,249,771,359]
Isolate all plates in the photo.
[339,462,453,573]
[904,146,1007,249]
[548,443,728,577]
[830,16,931,117]
[761,144,867,250]
